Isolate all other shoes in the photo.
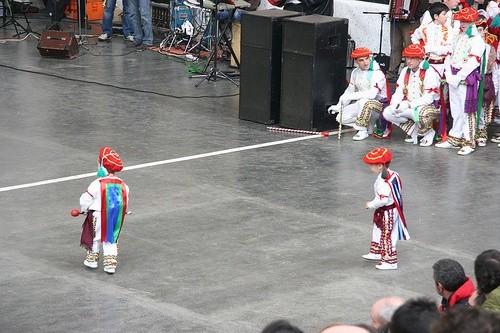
[478,143,486,147]
[123,36,134,41]
[435,141,460,149]
[136,44,148,51]
[457,146,474,156]
[98,34,111,40]
[420,130,435,146]
[353,131,369,140]
[84,259,98,268]
[362,253,381,260]
[491,136,500,143]
[376,261,397,270]
[128,41,136,48]
[104,265,117,273]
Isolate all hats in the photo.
[97,146,124,177]
[364,147,392,164]
[452,7,477,21]
[351,47,371,58]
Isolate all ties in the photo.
[403,44,429,69]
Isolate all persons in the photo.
[362,147,410,270]
[127,0,153,50]
[328,46,388,140]
[370,297,405,329]
[320,323,378,333]
[261,319,303,333]
[385,0,429,85]
[79,145,130,274]
[434,6,486,156]
[432,259,476,317]
[384,43,441,146]
[97,0,135,42]
[448,308,500,333]
[420,0,459,28]
[42,0,92,31]
[411,2,453,104]
[468,249,500,314]
[475,0,500,147]
[390,296,447,333]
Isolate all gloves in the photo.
[450,75,460,88]
[391,102,409,117]
[339,94,351,105]
[327,105,340,114]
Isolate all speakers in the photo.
[238,9,301,126]
[279,14,349,133]
[36,30,80,59]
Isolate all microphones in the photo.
[400,8,410,14]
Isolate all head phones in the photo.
[98,152,118,178]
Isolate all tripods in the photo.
[195,0,239,88]
